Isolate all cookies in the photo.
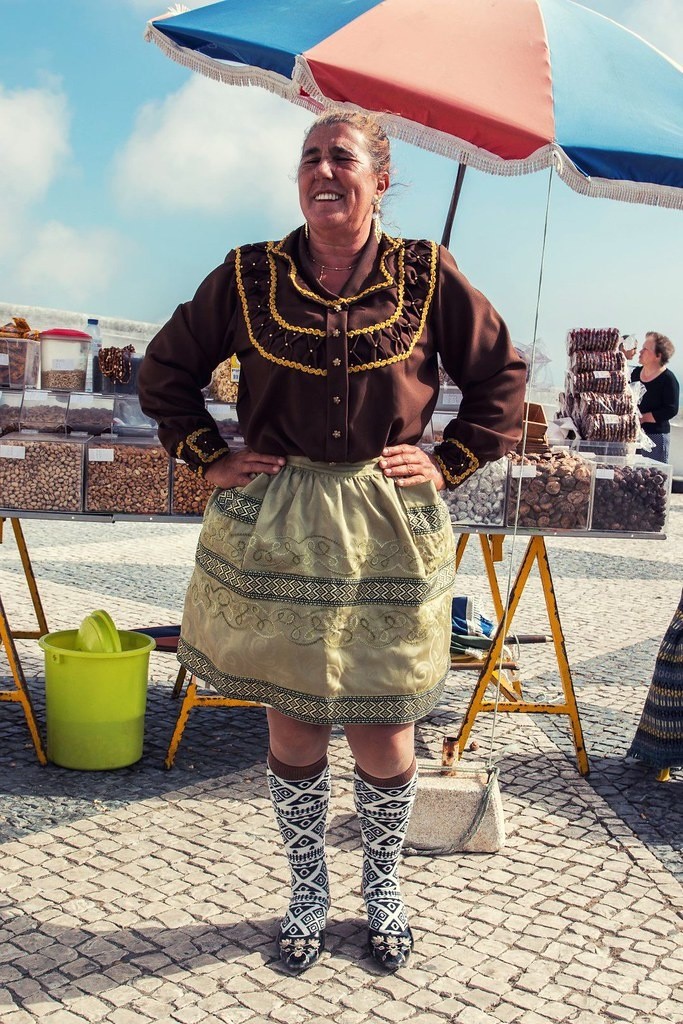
[552,328,637,442]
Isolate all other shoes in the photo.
[369,925,413,968]
[275,927,326,970]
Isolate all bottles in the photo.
[81,318,102,392]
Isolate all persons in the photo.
[619,335,638,360]
[626,332,678,464]
[133,108,531,969]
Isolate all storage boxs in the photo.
[0,329,672,532]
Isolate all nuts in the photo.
[0,404,217,514]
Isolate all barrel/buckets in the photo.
[37,610,156,768]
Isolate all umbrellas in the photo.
[142,0,683,250]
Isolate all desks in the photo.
[0,509,666,776]
[164,651,519,769]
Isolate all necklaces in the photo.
[306,252,357,280]
